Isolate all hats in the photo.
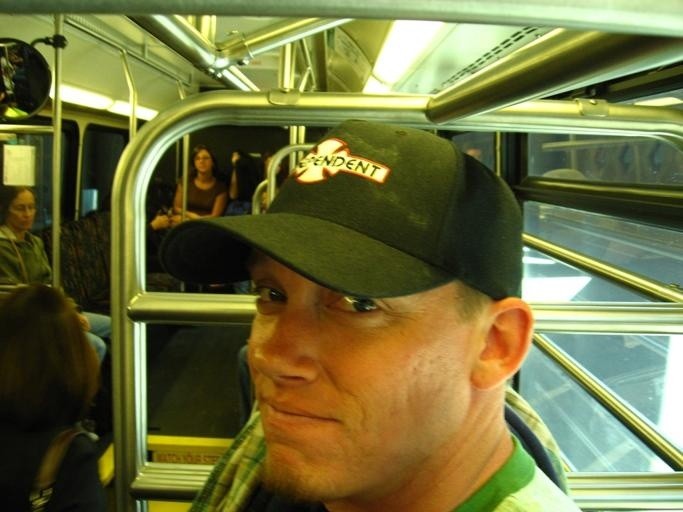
[158,117,525,303]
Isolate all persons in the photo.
[0,284,104,511]
[1,187,110,367]
[144,143,284,291]
[157,115,584,512]
